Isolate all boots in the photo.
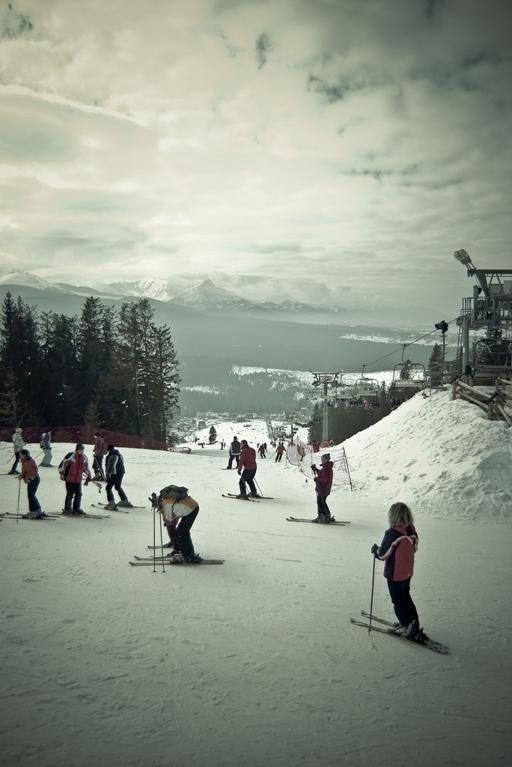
[104,500,117,510]
[24,509,42,518]
[117,499,129,507]
[247,490,258,497]
[237,491,248,499]
[313,513,331,523]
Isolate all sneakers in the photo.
[164,543,195,563]
[236,465,239,468]
[388,620,419,636]
[227,466,233,469]
[63,507,85,515]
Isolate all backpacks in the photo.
[160,484,188,499]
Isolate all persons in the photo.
[9,426,26,472]
[226,436,240,468]
[237,439,258,498]
[163,513,180,556]
[59,441,91,514]
[151,491,200,563]
[256,438,336,462]
[105,444,129,507]
[18,448,43,517]
[371,502,418,636]
[39,429,52,466]
[92,432,106,479]
[311,453,333,523]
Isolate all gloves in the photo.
[237,469,242,475]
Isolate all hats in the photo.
[320,453,331,461]
[16,427,23,433]
[95,432,101,437]
[106,444,115,450]
[151,492,159,506]
[76,442,83,450]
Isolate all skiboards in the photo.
[92,502,146,513]
[350,610,449,655]
[48,508,111,519]
[285,516,350,526]
[0,512,58,520]
[129,555,223,566]
[148,544,177,558]
[222,492,275,502]
